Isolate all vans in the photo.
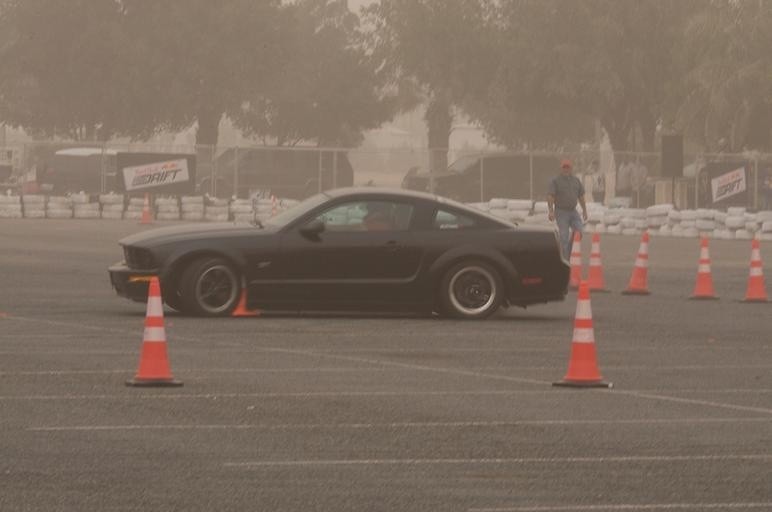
[197,146,355,203]
[405,151,566,203]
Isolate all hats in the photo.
[562,159,572,168]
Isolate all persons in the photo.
[361,201,395,233]
[545,159,591,263]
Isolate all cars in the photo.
[109,187,570,323]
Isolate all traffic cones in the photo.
[689,236,717,299]
[620,232,651,295]
[232,277,259,316]
[744,236,767,303]
[567,231,586,290]
[139,194,155,224]
[586,233,604,292]
[552,282,614,389]
[127,277,183,390]
